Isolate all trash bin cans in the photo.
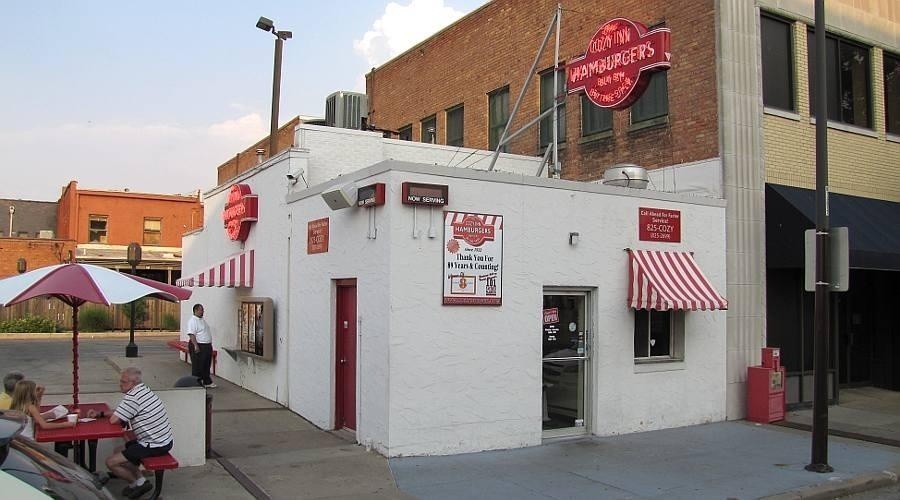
[173,376,213,458]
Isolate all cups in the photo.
[66,413,78,424]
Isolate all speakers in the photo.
[320,181,358,210]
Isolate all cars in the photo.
[0,408,119,500]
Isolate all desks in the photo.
[33,401,125,474]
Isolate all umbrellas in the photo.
[0,260,194,415]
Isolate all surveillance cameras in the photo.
[286,168,304,180]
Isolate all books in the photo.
[40,405,69,422]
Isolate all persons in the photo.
[186,303,218,389]
[0,370,45,414]
[10,379,76,440]
[105,366,175,500]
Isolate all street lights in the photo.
[16,256,29,323]
[255,16,294,163]
[124,242,141,359]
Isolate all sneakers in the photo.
[203,383,216,388]
[122,480,152,499]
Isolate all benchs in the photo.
[167,340,217,376]
[126,431,178,498]
